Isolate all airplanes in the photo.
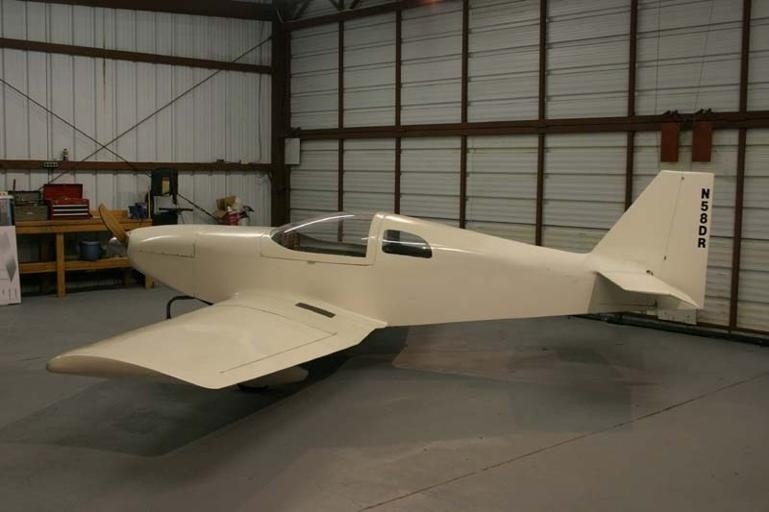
[45,169,716,394]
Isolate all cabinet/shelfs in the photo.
[1,212,154,298]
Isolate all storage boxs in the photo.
[11,191,45,220]
[0,192,15,226]
[45,183,91,217]
[215,196,251,225]
